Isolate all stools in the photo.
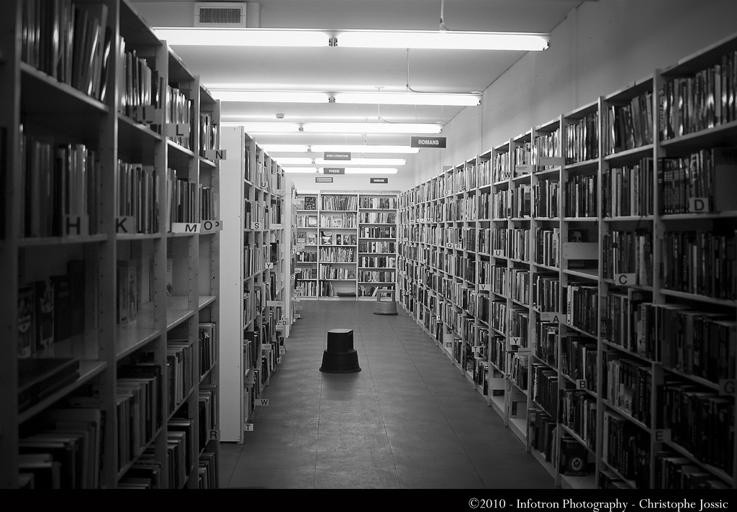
[319,329,362,373]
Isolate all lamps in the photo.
[149,0,550,175]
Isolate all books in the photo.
[20,0,217,492]
[245,135,396,425]
[395,53,736,488]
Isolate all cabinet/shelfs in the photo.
[401,30,736,488]
[0,0,220,491]
[295,194,400,300]
[220,126,295,444]
